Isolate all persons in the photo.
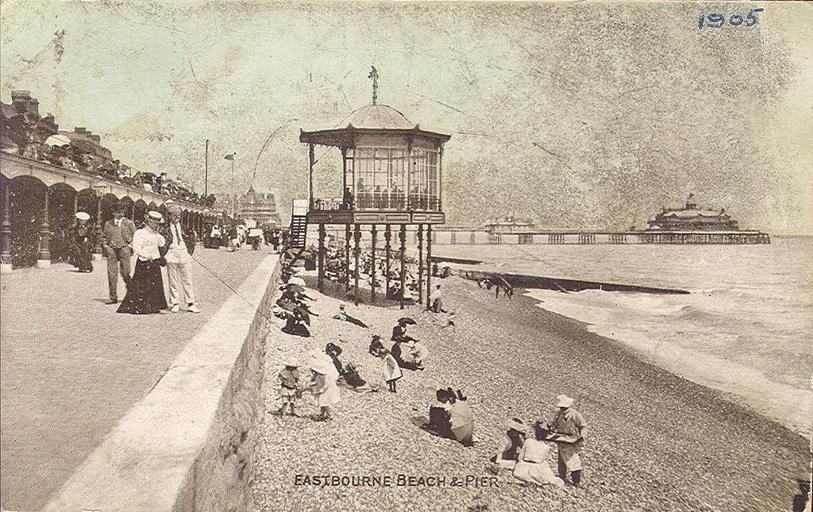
[10,119,585,488]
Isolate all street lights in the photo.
[223,154,234,219]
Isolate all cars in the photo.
[203,222,282,249]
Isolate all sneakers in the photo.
[105,298,118,305]
[172,304,179,313]
[187,305,201,314]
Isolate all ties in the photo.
[174,224,182,246]
[116,221,119,227]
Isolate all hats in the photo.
[310,359,329,375]
[282,357,303,368]
[143,210,164,223]
[75,212,91,220]
[556,395,575,409]
